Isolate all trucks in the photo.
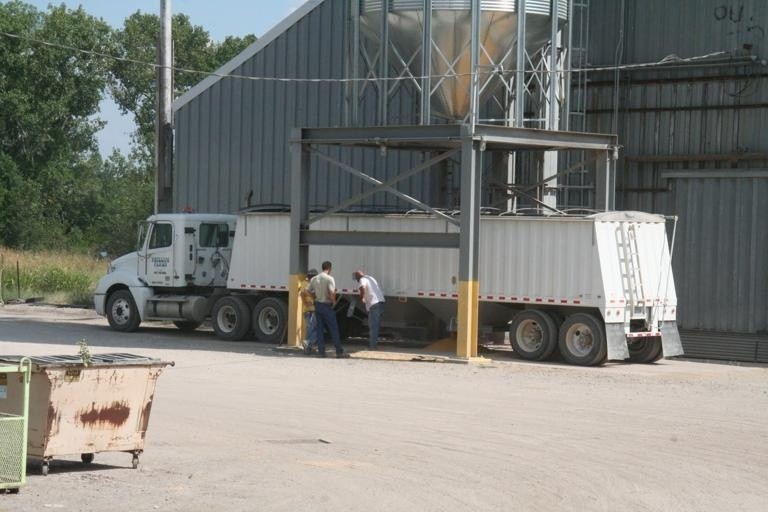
[93,189,686,369]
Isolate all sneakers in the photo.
[336,353,351,358]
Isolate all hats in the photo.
[306,269,318,275]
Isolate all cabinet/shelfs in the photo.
[0,351,32,497]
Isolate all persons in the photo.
[301,268,317,354]
[352,270,386,351]
[306,261,350,358]
